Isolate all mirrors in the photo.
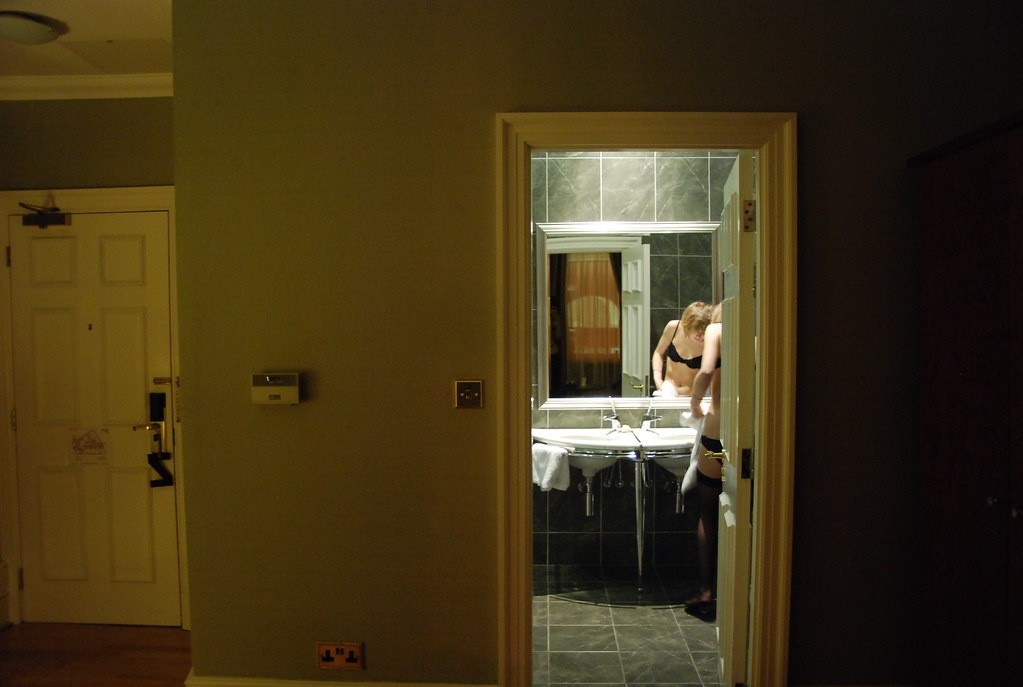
[535,220,721,409]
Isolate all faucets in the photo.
[603,414,621,430]
[640,413,663,429]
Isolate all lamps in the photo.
[0,14,57,46]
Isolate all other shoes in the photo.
[685,598,717,620]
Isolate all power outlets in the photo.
[316,640,363,672]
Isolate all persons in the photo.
[678,300,727,608]
[652,300,712,401]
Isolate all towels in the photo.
[531,443,571,492]
[680,402,712,494]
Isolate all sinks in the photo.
[532,427,698,450]
